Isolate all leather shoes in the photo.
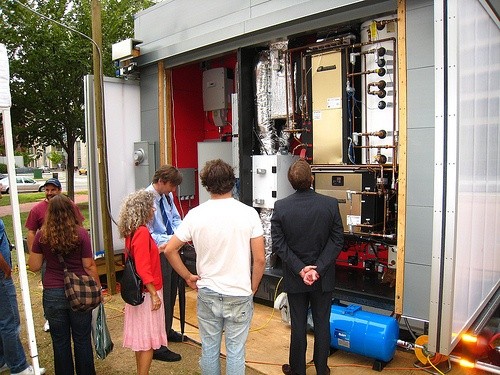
[152,345,181,361]
[167,328,188,341]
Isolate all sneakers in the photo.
[44,320,50,332]
[0,363,8,370]
[10,365,46,375]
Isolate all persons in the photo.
[0,182,46,374]
[144,165,189,362]
[164,160,266,375]
[25,178,83,332]
[118,191,167,375]
[271,159,345,375]
[28,195,105,375]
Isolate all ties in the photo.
[160,198,174,235]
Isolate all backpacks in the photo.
[115,225,147,306]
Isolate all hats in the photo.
[44,178,61,188]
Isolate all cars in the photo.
[0,176,46,194]
[79,167,87,176]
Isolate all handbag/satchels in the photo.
[91,302,115,360]
[64,272,100,313]
[179,244,197,287]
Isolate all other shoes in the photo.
[282,364,294,375]
[326,365,330,375]
